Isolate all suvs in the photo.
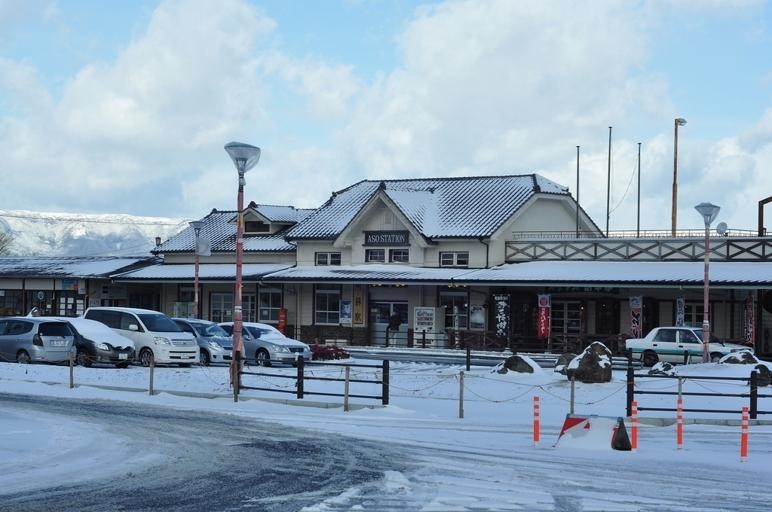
[0,316,77,367]
[53,317,136,369]
[171,317,247,368]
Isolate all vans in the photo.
[81,306,201,368]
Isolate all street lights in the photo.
[188,218,209,322]
[690,196,722,363]
[672,114,690,237]
[224,141,264,389]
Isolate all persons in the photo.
[386,308,402,347]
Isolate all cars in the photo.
[624,327,757,371]
[206,322,313,368]
